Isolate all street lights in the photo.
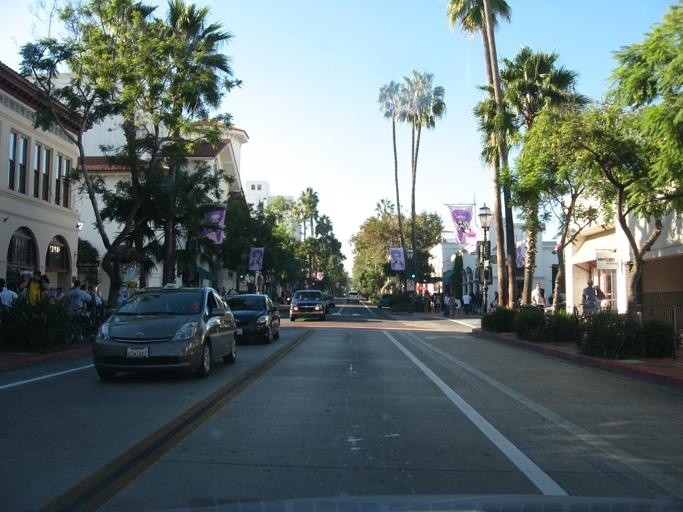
[477,204,492,313]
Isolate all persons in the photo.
[423,289,498,318]
[216,287,238,297]
[581,280,598,322]
[185,297,201,313]
[530,282,545,310]
[0,269,105,325]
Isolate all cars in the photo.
[348,291,360,303]
[93,284,237,377]
[225,294,280,343]
[290,290,335,320]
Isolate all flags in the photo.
[248,246,264,270]
[450,207,475,244]
[198,210,226,246]
[313,272,324,282]
[390,248,405,270]
[516,240,525,269]
